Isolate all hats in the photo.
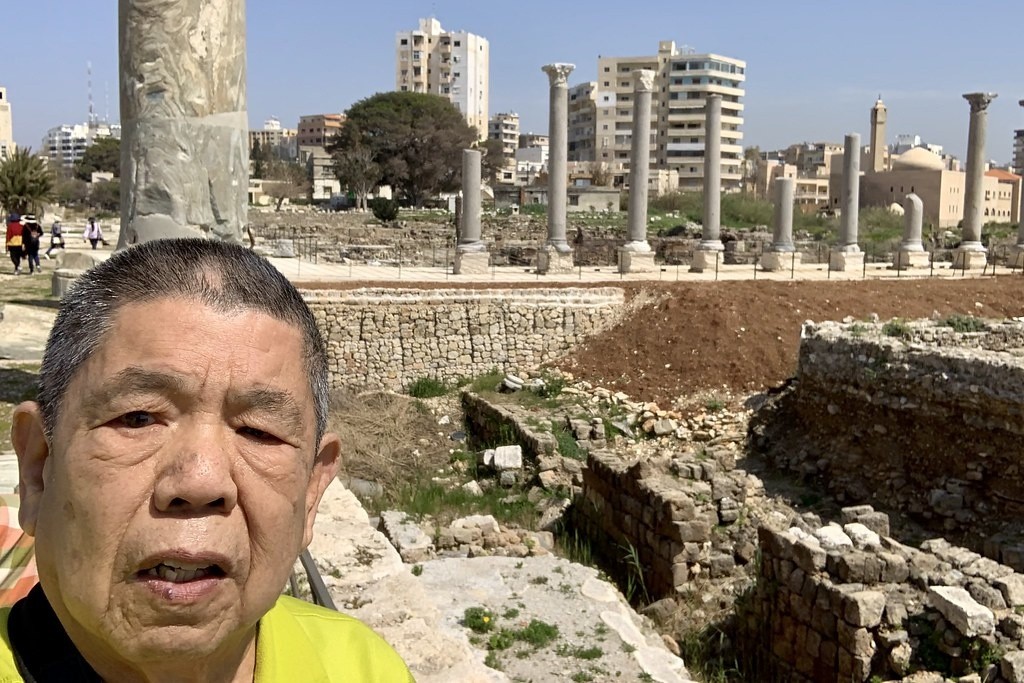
[8,213,22,221]
[19,213,38,223]
[54,216,62,222]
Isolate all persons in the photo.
[6,206,44,276]
[83,217,109,250]
[45,217,65,260]
[0,240,419,683]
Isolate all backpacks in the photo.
[27,223,40,240]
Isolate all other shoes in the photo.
[36,265,41,272]
[14,270,21,275]
[44,253,51,260]
[104,242,110,246]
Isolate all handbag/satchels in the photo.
[52,235,64,244]
[97,224,103,241]
